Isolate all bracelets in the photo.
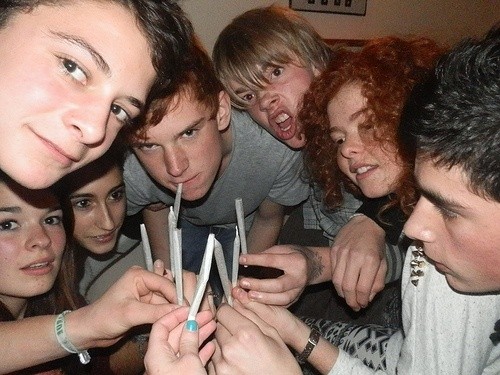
[293,323,322,365]
[55,309,92,365]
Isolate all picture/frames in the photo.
[288,0,367,16]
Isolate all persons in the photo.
[212,4,364,307]
[145,296,217,375]
[231,16,499,374]
[0,0,190,375]
[206,299,303,375]
[63,148,148,306]
[294,31,449,313]
[120,31,312,310]
[0,167,81,375]
